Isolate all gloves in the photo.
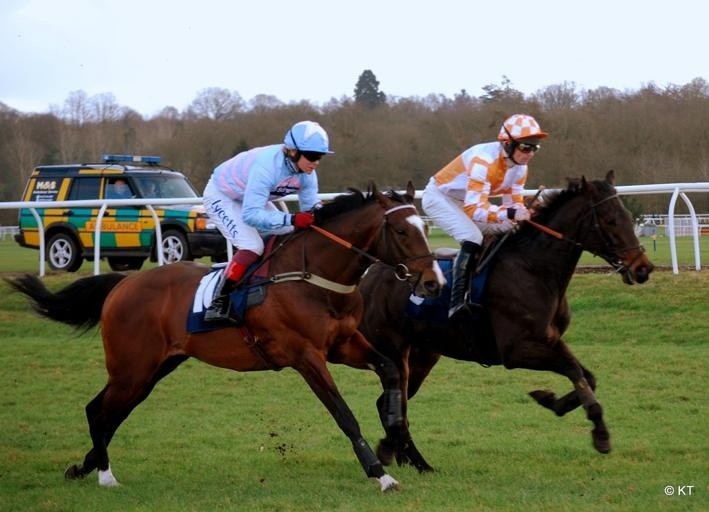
[291,212,314,228]
[507,207,532,221]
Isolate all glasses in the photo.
[510,141,541,153]
[297,149,326,162]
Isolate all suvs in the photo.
[15,155,238,271]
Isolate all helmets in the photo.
[284,120,334,154]
[497,113,549,141]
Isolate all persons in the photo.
[111,181,135,199]
[147,182,160,198]
[422,112,552,318]
[204,121,336,325]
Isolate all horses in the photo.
[1,178,453,496]
[360,169,656,468]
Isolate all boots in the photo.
[204,260,247,321]
[448,240,483,321]
